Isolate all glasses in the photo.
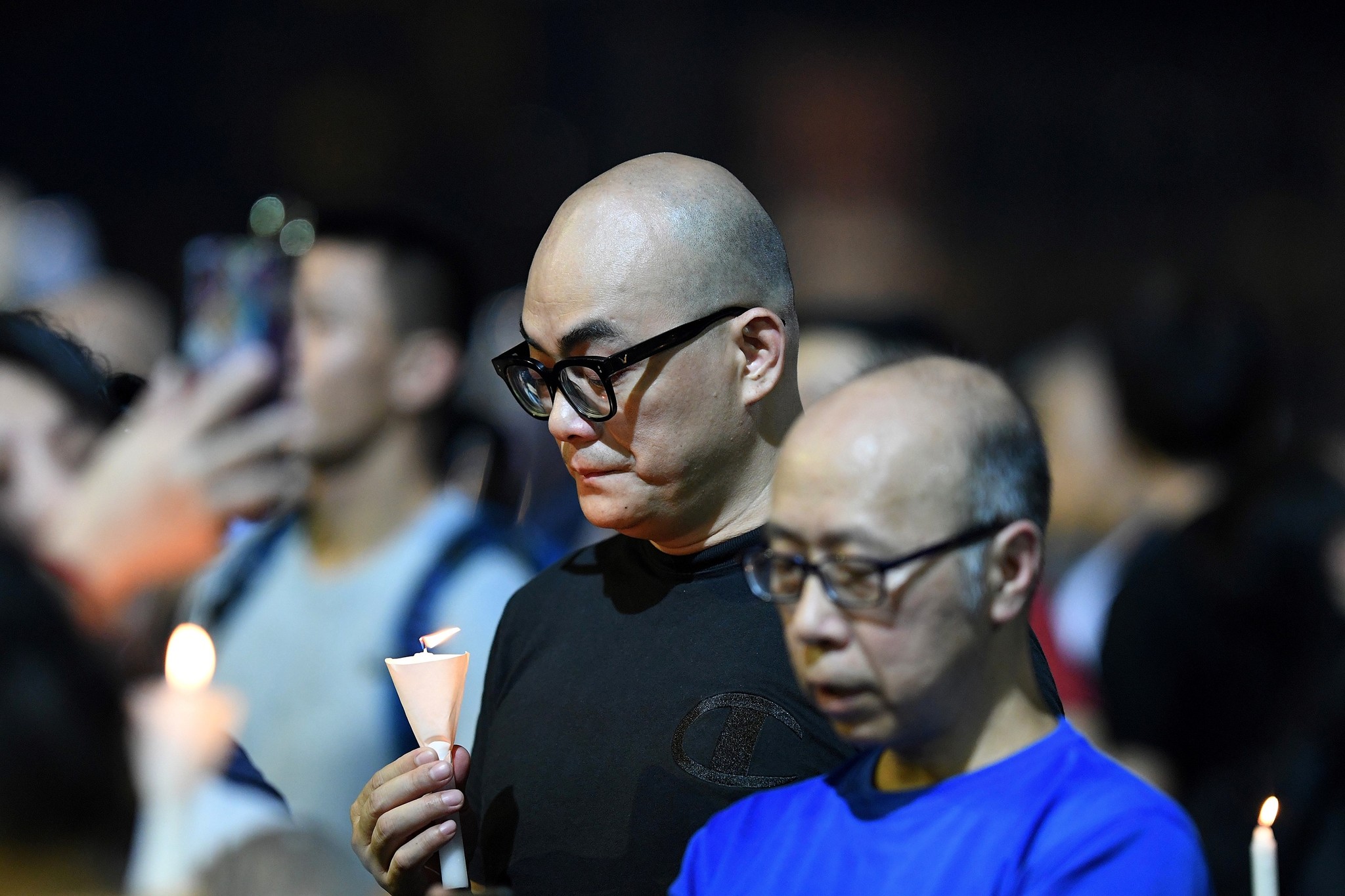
[492,301,753,425]
[742,518,1000,610]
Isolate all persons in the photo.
[1,153,1344,896]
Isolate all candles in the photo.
[1250,796,1279,896]
[413,627,469,889]
